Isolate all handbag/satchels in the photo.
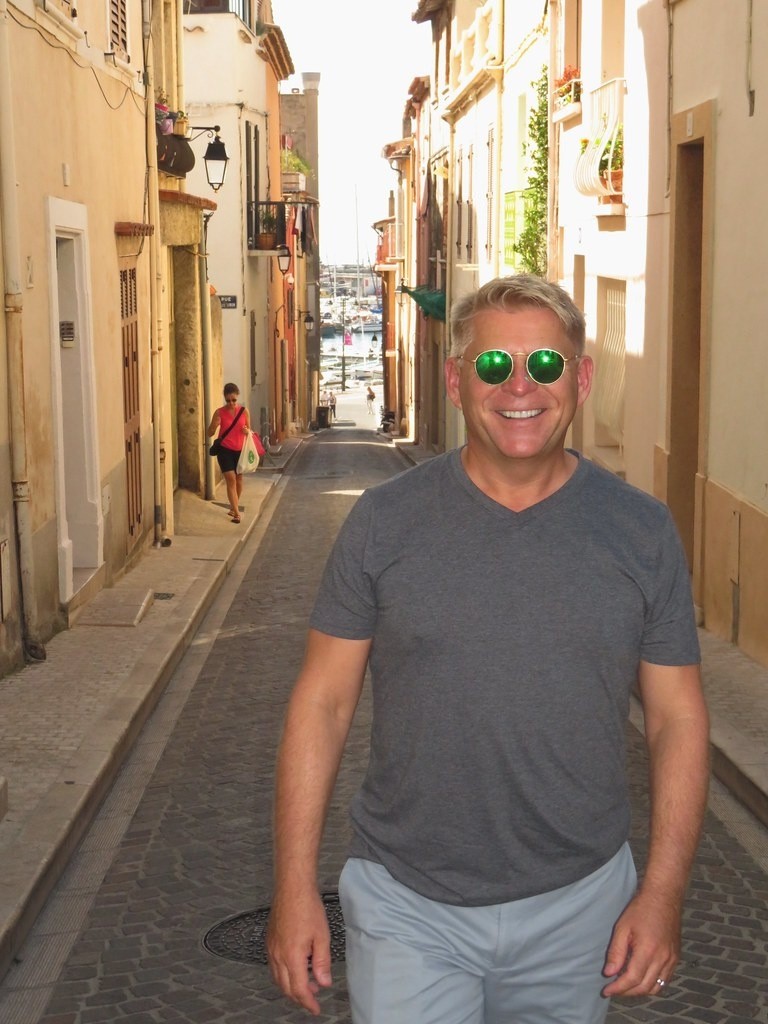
[210,439,221,456]
[236,430,266,474]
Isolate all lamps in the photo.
[189,125,230,193]
[394,277,414,306]
[270,246,292,282]
[298,310,314,332]
[371,330,384,348]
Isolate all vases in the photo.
[597,169,623,203]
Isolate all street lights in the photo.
[339,297,348,391]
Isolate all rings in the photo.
[656,978,665,986]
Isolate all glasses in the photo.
[226,399,236,402]
[457,349,581,386]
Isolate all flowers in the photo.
[553,64,580,103]
[580,115,623,169]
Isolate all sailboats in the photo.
[320,183,384,317]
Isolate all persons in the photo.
[321,391,328,400]
[329,391,337,418]
[208,383,251,523]
[267,272,710,1024]
[366,386,375,414]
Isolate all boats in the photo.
[320,316,384,386]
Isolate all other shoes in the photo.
[231,515,241,523]
[228,509,235,516]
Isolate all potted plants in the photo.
[154,85,173,136]
[258,211,277,250]
[281,150,311,193]
